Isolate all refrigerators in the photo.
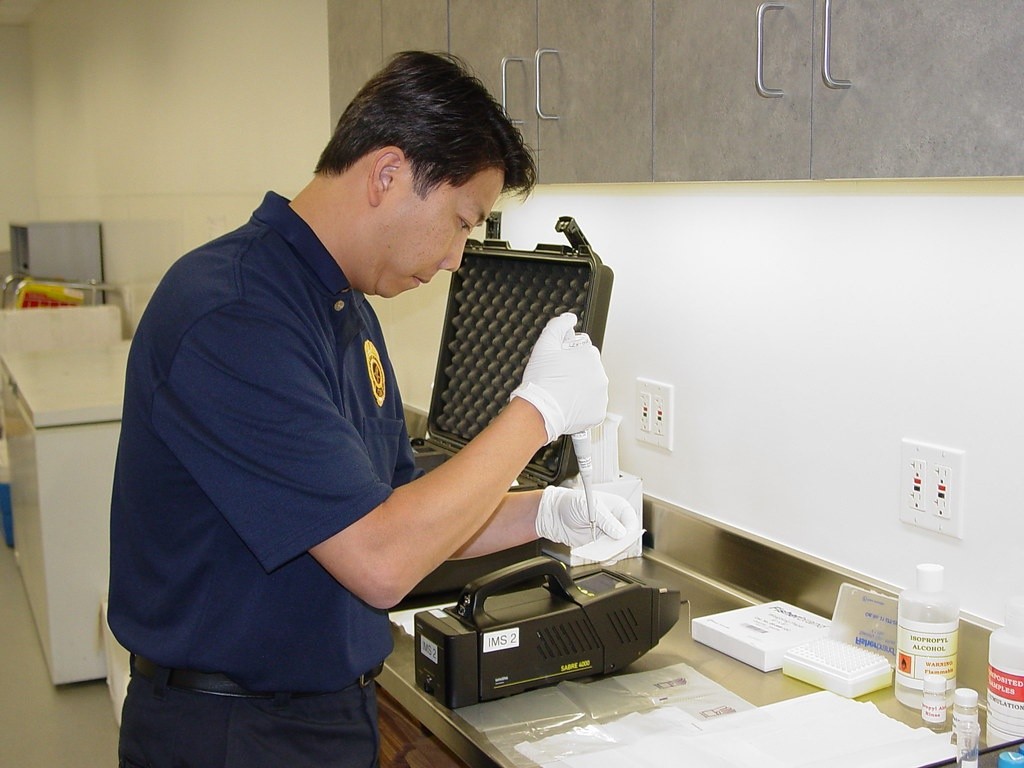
[0,340,133,686]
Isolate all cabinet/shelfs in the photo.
[327,0,449,137]
[448,0,653,184]
[654,0,1024,183]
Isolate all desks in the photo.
[375,549,989,768]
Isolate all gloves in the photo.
[510,312,609,446]
[535,486,639,566]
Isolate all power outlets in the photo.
[635,378,675,452]
[899,438,968,540]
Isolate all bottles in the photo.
[922,675,946,731]
[988,604,1024,747]
[895,563,959,711]
[952,689,979,740]
[957,719,980,768]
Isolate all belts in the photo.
[134,655,384,697]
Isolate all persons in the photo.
[106,50,610,768]
